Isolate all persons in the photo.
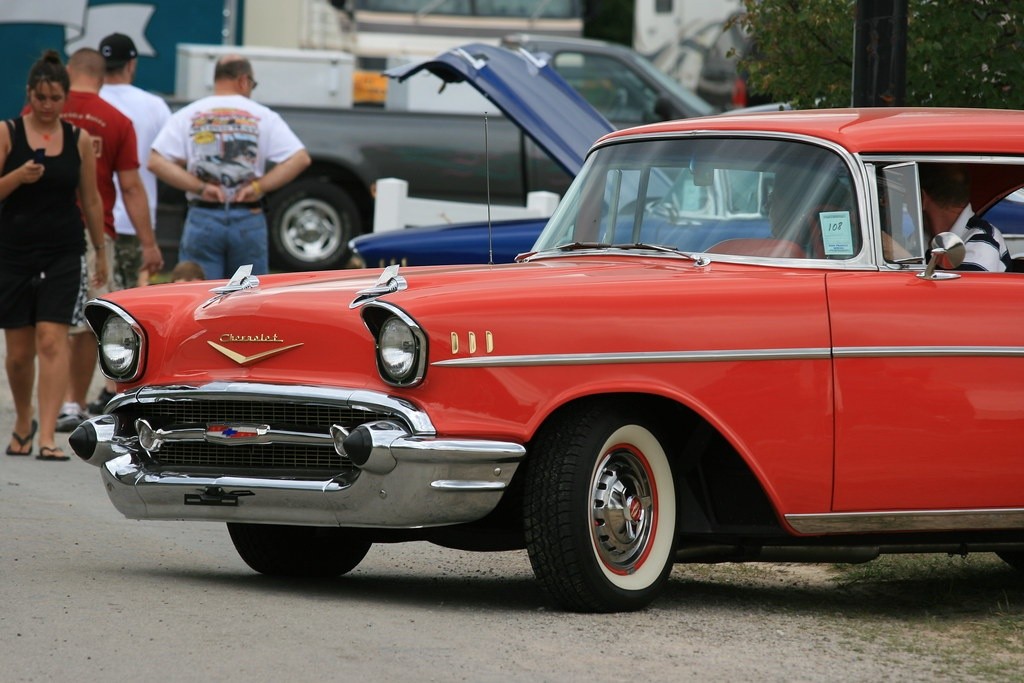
[147,55,312,280]
[769,163,1013,273]
[0,49,109,462]
[24,31,170,426]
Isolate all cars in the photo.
[67,107,1023,615]
[346,41,1023,268]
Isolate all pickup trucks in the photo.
[151,35,718,272]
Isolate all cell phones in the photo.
[33,148,44,163]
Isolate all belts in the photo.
[190,199,261,209]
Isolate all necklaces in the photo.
[36,129,57,142]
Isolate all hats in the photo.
[99,32,137,63]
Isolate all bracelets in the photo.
[251,181,261,197]
[94,244,107,250]
[197,181,206,193]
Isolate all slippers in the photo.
[6,419,38,456]
[35,447,70,461]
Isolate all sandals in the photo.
[87,386,117,415]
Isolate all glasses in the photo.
[247,77,257,89]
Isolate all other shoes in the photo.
[55,411,82,432]
